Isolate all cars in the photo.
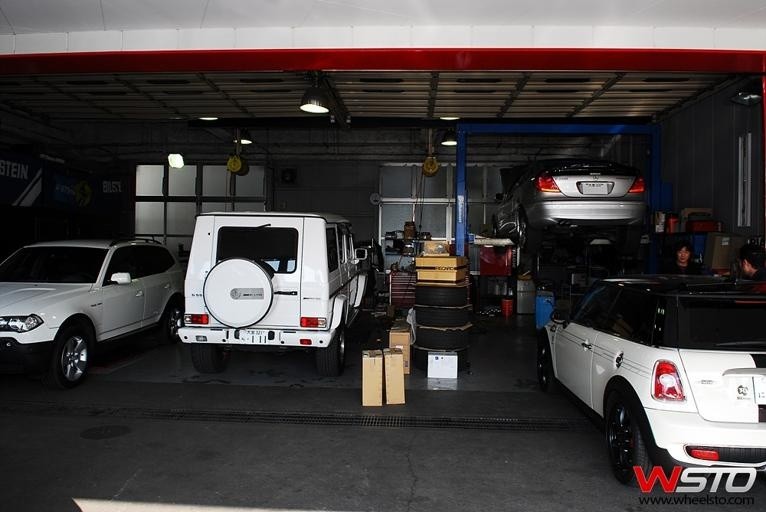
[491,157,653,249]
[532,270,766,493]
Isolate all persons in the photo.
[662,241,701,274]
[738,244,766,282]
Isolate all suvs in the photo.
[173,208,368,381]
[0,231,185,393]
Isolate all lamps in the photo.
[297,69,334,116]
[232,131,252,146]
[438,132,459,148]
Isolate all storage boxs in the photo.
[415,257,467,283]
[426,352,458,380]
[423,240,450,257]
[361,322,412,408]
